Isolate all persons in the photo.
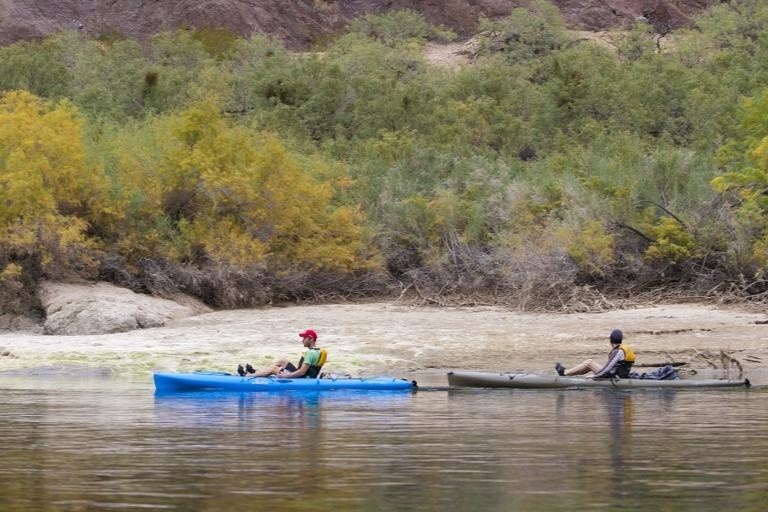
[555,329,635,379]
[237,330,320,378]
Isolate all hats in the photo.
[298,329,318,339]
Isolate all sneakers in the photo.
[245,362,256,374]
[237,364,247,377]
[555,362,566,377]
